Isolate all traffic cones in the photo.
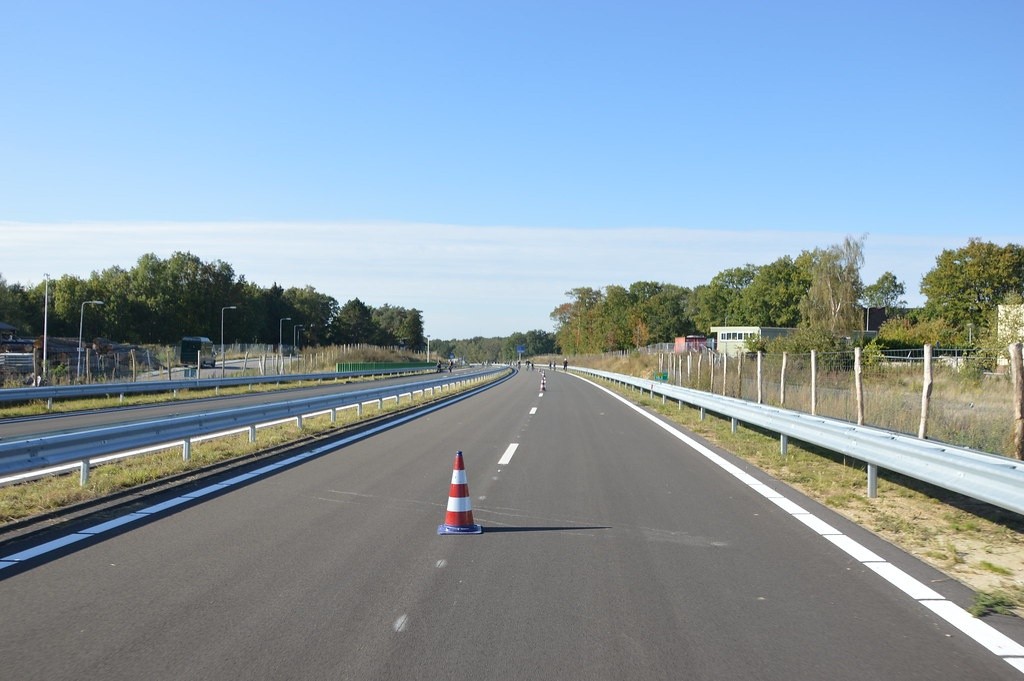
[433,453,482,536]
[540,369,547,390]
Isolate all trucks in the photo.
[180,340,217,367]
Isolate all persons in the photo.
[435,357,454,373]
[491,358,569,371]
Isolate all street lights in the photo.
[220,306,237,377]
[73,301,104,382]
[280,317,291,367]
[294,324,305,353]
